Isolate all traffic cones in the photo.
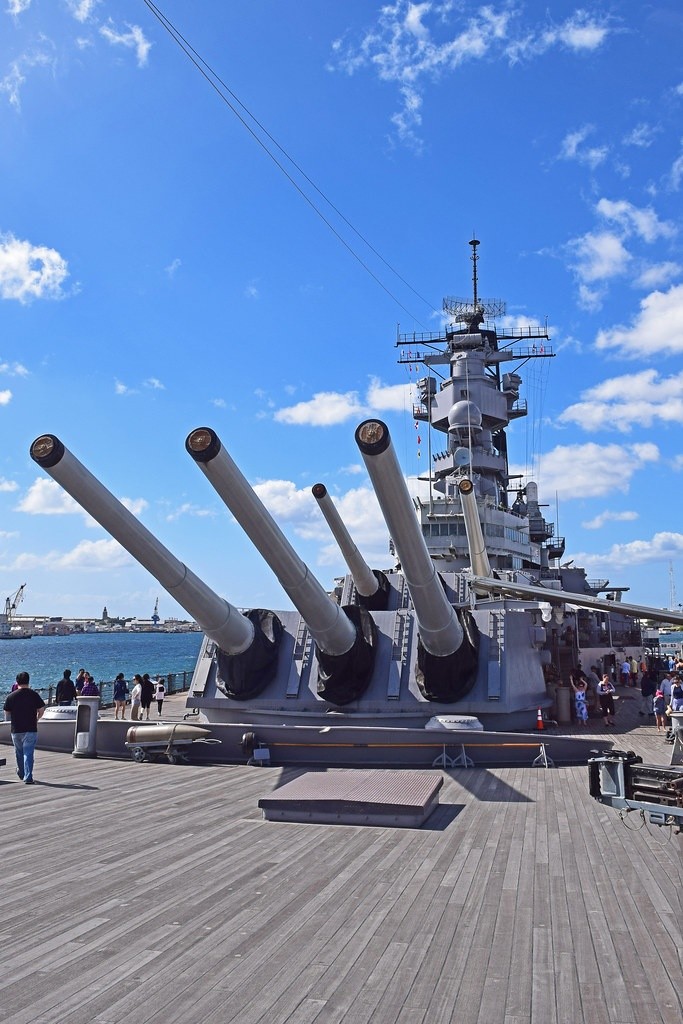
[533,706,547,730]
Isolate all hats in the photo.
[655,689,664,698]
[576,663,583,667]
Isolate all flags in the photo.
[408,351,421,458]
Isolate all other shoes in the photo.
[147,718,150,720]
[16,768,24,780]
[658,728,661,732]
[139,713,143,721]
[663,728,666,732]
[648,712,654,716]
[625,685,629,688]
[121,717,126,720]
[115,717,119,720]
[606,724,610,727]
[608,721,615,726]
[25,779,35,784]
[639,710,645,715]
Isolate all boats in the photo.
[0,583,33,639]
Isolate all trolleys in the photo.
[124,738,222,764]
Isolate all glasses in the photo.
[674,682,679,684]
[578,686,584,687]
[133,679,135,682]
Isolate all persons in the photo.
[639,672,654,716]
[653,690,666,732]
[156,679,166,715]
[659,673,683,711]
[622,656,638,687]
[113,673,130,720]
[3,671,46,783]
[576,664,600,690]
[596,674,615,726]
[139,674,154,720]
[653,656,683,671]
[130,674,142,720]
[569,675,588,725]
[55,669,78,706]
[75,669,99,696]
